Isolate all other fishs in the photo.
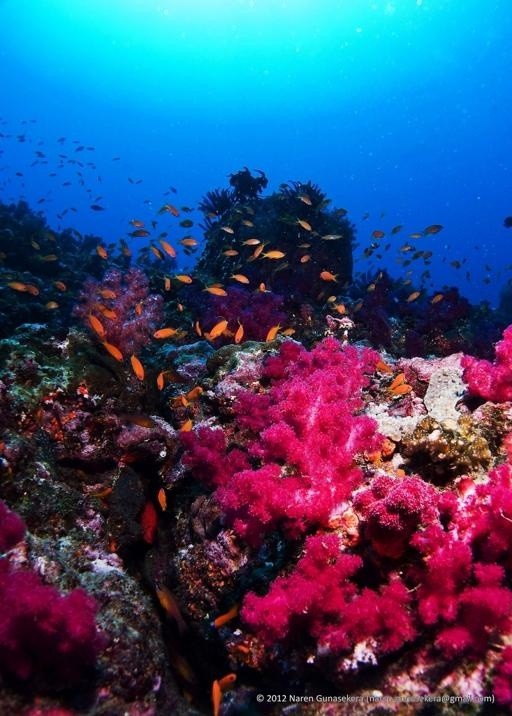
[0,118,511,406]
